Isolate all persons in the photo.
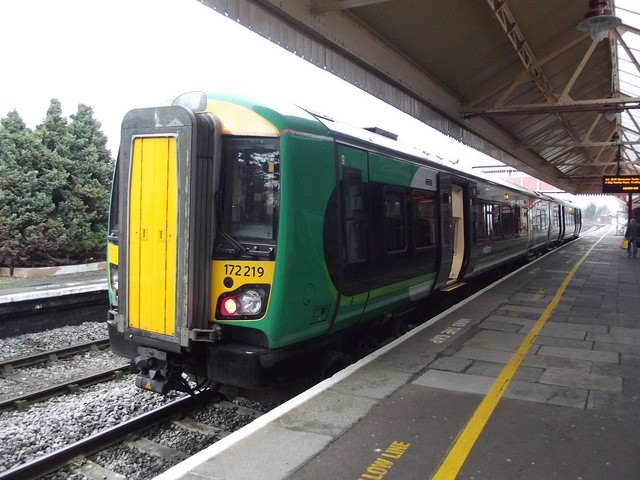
[625,220,640,258]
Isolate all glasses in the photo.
[575,1,622,42]
[611,166,626,174]
[611,151,624,162]
[610,131,619,142]
[599,68,624,120]
